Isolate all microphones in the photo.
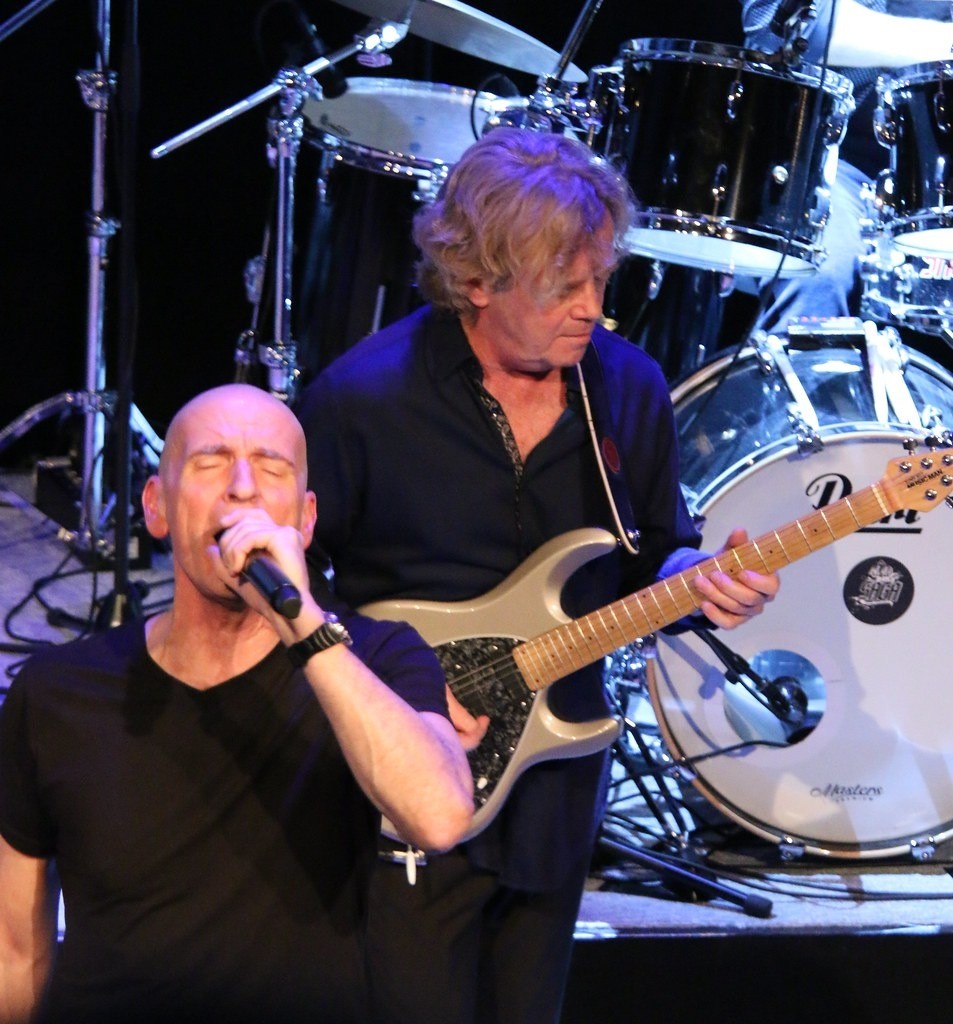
[770,675,810,725]
[235,546,301,620]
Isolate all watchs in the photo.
[288,610,354,672]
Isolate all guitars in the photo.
[333,441,952,844]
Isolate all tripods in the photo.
[0,0,180,637]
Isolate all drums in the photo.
[294,36,953,857]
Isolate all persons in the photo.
[0,385,478,1023]
[294,124,781,1023]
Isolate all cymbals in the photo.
[351,1,598,79]
